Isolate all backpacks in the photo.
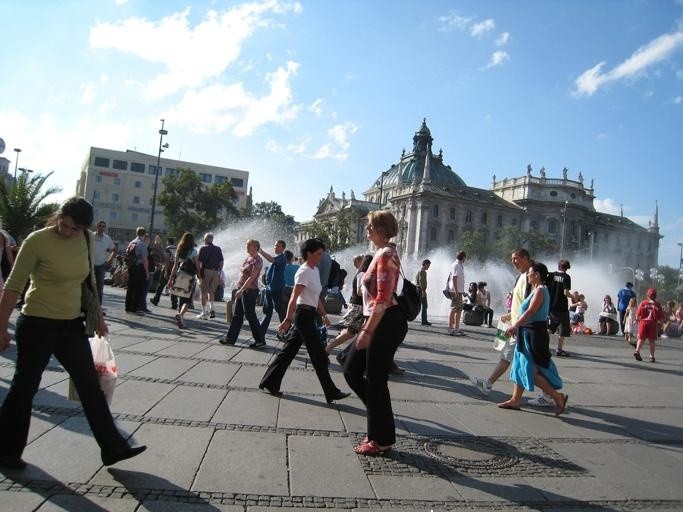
[124,241,140,267]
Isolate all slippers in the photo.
[361,434,369,444]
[351,442,392,455]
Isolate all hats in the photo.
[646,287,656,299]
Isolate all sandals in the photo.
[495,401,520,410]
[648,357,655,362]
[556,349,569,357]
[554,390,569,416]
[632,350,642,361]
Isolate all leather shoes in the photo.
[325,388,351,404]
[257,382,283,397]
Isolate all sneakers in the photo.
[209,309,215,319]
[452,330,465,336]
[218,338,234,347]
[195,312,208,320]
[98,443,148,468]
[175,313,184,329]
[422,321,432,326]
[249,340,266,348]
[484,324,488,328]
[447,328,454,335]
[140,307,151,315]
[488,324,496,328]
[149,297,157,306]
[468,374,492,396]
[526,393,553,408]
[0,455,27,470]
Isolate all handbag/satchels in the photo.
[178,258,198,277]
[66,334,119,408]
[392,275,423,322]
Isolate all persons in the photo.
[416,259,433,325]
[598,282,682,344]
[448,251,466,336]
[219,237,405,403]
[462,281,495,328]
[0,218,225,328]
[472,247,588,417]
[342,211,409,454]
[0,197,147,470]
[633,288,665,362]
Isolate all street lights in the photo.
[558,199,570,259]
[10,147,22,184]
[633,267,646,301]
[16,167,33,179]
[587,232,594,266]
[649,265,666,304]
[146,117,169,245]
[375,170,390,212]
